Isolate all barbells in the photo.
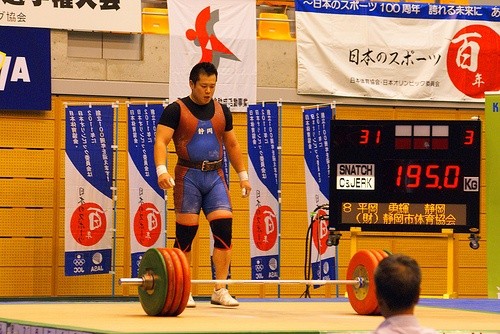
[117,248,394,316]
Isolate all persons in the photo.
[366,255,436,334]
[153,62,252,306]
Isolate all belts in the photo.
[176,158,223,172]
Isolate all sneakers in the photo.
[186,292,196,308]
[211,288,239,306]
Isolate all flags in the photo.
[166,0,261,115]
